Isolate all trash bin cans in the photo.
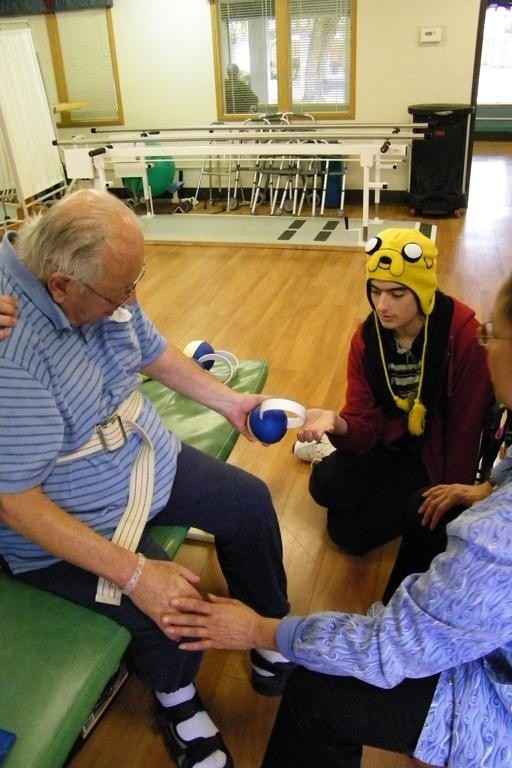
[408,103,476,219]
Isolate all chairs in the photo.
[188,111,349,219]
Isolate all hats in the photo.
[365,230,438,315]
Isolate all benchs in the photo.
[0,358,270,768]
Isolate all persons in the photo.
[297,229,499,608]
[0,191,295,761]
[224,62,259,114]
[0,293,18,343]
[158,273,511,767]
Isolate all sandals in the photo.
[250,646,294,697]
[154,680,234,768]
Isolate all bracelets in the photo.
[117,551,145,594]
[487,477,500,490]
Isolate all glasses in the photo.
[74,261,147,307]
[477,321,511,345]
